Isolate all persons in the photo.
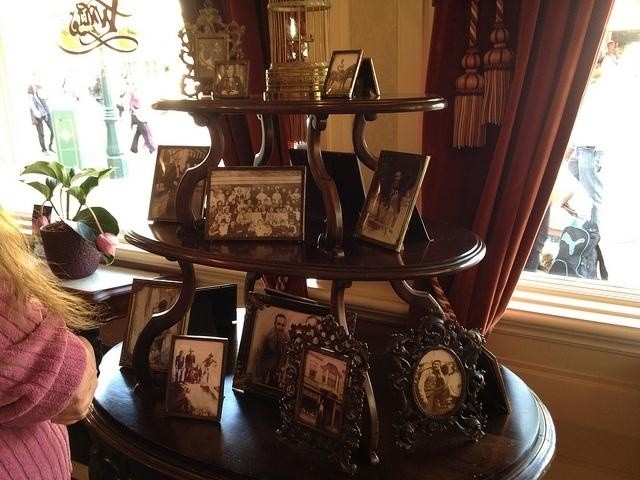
[162,149,187,218]
[526,52,609,284]
[171,346,218,417]
[0,213,100,480]
[381,169,407,214]
[198,41,243,97]
[210,171,302,237]
[419,356,455,417]
[249,312,293,391]
[336,57,349,81]
[144,297,170,361]
[313,397,326,427]
[25,68,158,155]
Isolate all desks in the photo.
[55,258,163,371]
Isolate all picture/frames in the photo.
[385,306,490,457]
[238,284,369,471]
[320,47,366,99]
[146,139,308,246]
[176,0,250,99]
[124,271,226,423]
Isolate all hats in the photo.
[188,348,194,352]
[209,353,213,357]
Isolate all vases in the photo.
[42,219,101,278]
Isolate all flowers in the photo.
[19,158,122,260]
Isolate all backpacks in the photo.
[547,226,608,280]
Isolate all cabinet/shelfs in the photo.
[79,96,556,474]
